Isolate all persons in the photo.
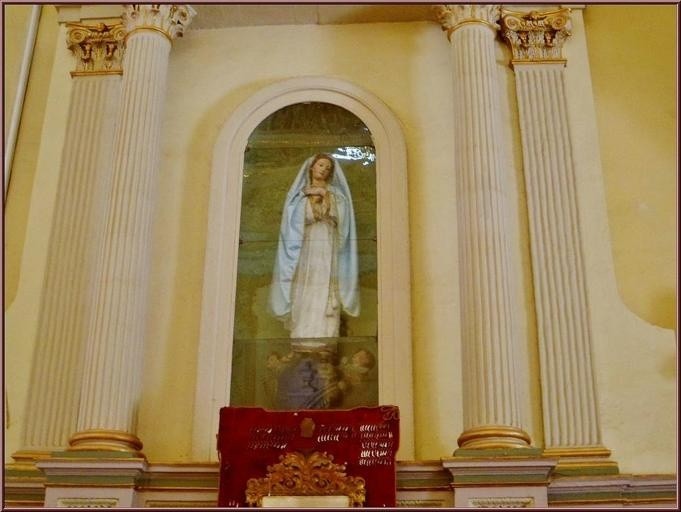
[269,153,361,346]
